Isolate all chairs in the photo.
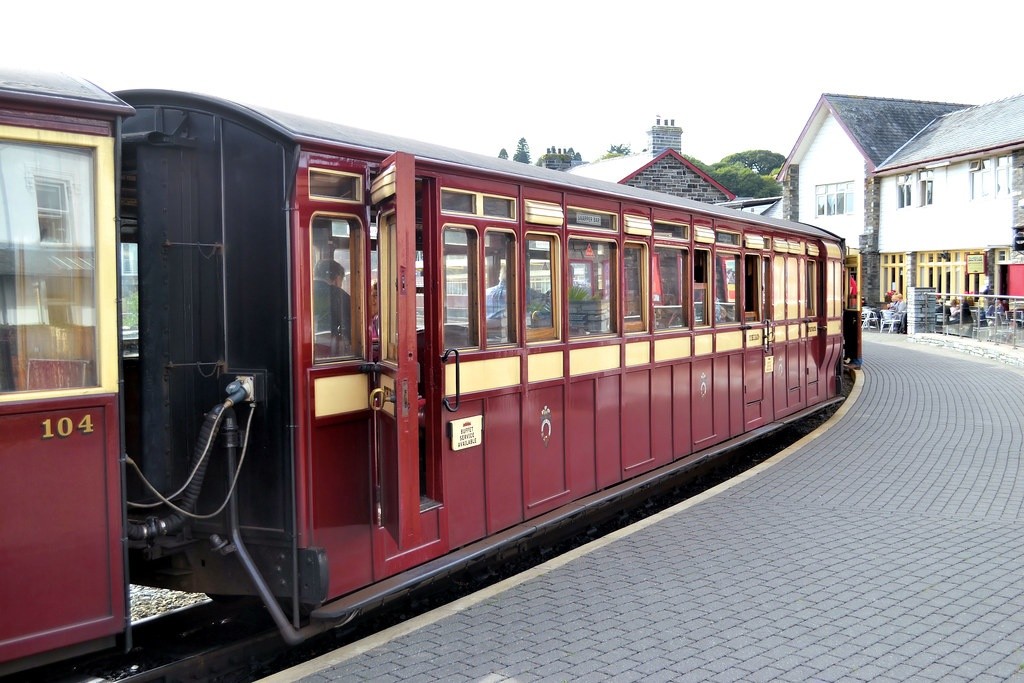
[860,304,1024,348]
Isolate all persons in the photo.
[843,269,862,370]
[987,300,1004,339]
[485,265,544,319]
[949,299,960,323]
[962,303,973,323]
[886,294,906,311]
[369,279,379,344]
[1002,300,1009,312]
[976,305,988,327]
[936,298,951,323]
[313,258,350,340]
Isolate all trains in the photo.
[0,70,861,683]
